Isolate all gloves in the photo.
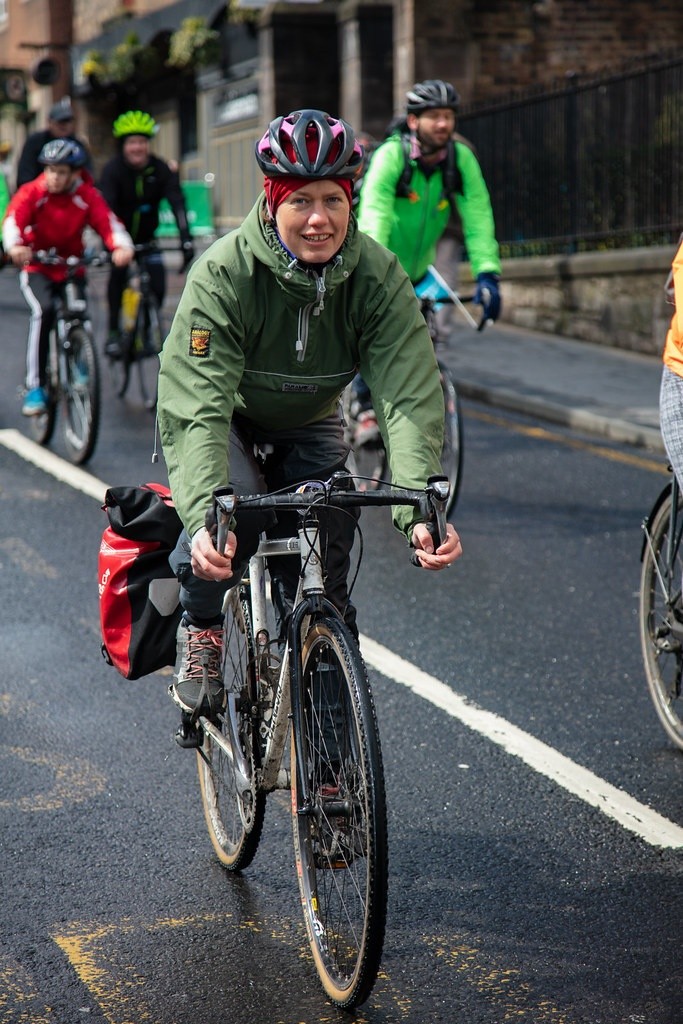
[181,234,194,258]
[473,277,502,322]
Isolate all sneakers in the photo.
[309,776,356,869]
[172,614,226,717]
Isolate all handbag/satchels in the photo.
[97,482,179,680]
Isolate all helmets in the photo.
[112,111,157,141]
[256,108,365,176]
[405,81,460,111]
[38,140,88,170]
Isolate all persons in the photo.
[351,79,503,448]
[17,94,95,190]
[659,232,683,490]
[3,139,136,416]
[0,164,11,263]
[97,110,195,355]
[157,112,462,859]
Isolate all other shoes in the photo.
[19,388,46,414]
[105,333,122,357]
[354,411,382,448]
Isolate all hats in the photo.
[47,105,76,123]
[264,135,353,212]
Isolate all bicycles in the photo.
[339,278,492,523]
[173,470,450,1006]
[637,463,683,755]
[89,242,194,412]
[0,245,144,465]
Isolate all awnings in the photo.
[72,0,231,95]
